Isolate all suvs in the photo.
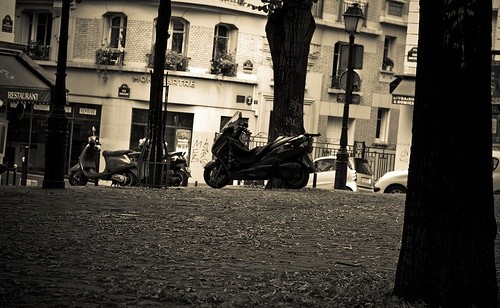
[306,155,375,192]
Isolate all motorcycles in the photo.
[132,138,192,187]
[203,111,323,189]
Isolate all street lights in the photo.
[333,1,367,190]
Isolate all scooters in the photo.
[69,126,138,186]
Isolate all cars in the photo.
[373,170,408,193]
[491,150,500,193]
